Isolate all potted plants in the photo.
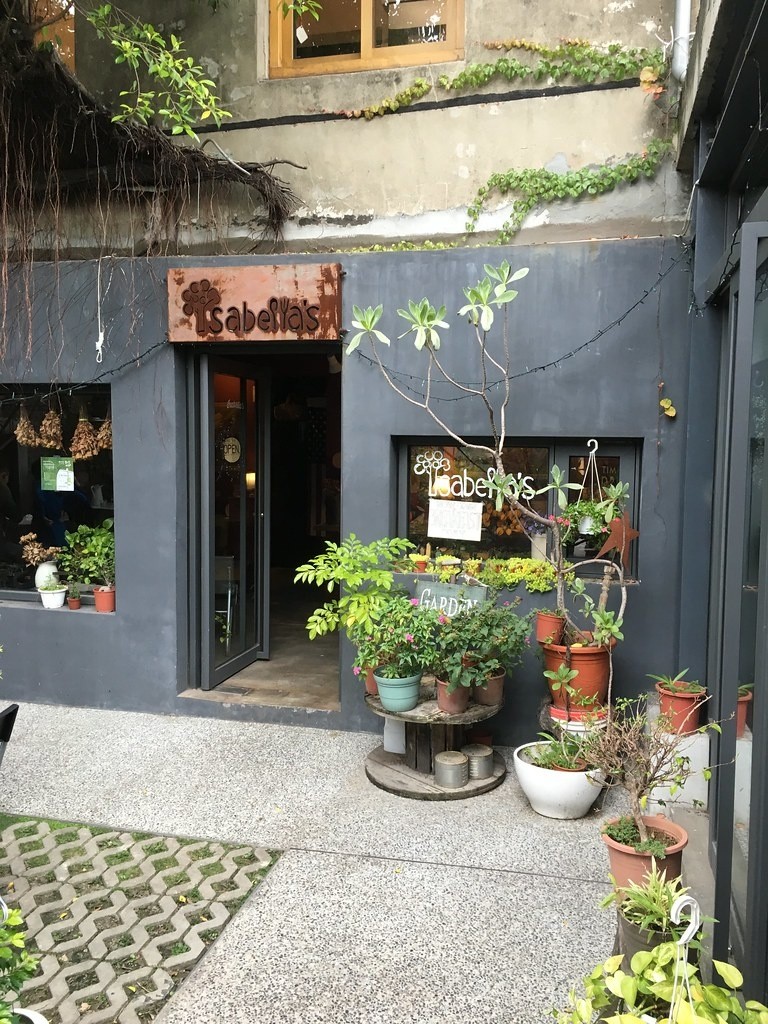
[737,683,755,740]
[38,576,67,608]
[293,533,417,695]
[487,559,504,573]
[506,558,522,573]
[537,732,587,772]
[581,694,737,905]
[346,260,618,711]
[72,518,116,613]
[394,555,411,573]
[534,607,570,643]
[543,942,768,1024]
[613,871,705,978]
[57,546,82,610]
[463,556,483,573]
[412,553,429,573]
[513,668,608,820]
[643,668,706,735]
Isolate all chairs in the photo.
[215,556,239,657]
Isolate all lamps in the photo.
[430,475,456,501]
[246,472,256,498]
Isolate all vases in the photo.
[371,664,425,712]
[35,561,59,589]
[434,674,472,713]
[470,666,505,705]
[461,656,481,669]
[576,515,602,535]
[530,534,566,562]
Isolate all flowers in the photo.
[483,501,525,535]
[434,590,509,693]
[524,512,566,535]
[548,499,620,546]
[471,596,535,674]
[350,596,448,681]
[18,532,61,566]
